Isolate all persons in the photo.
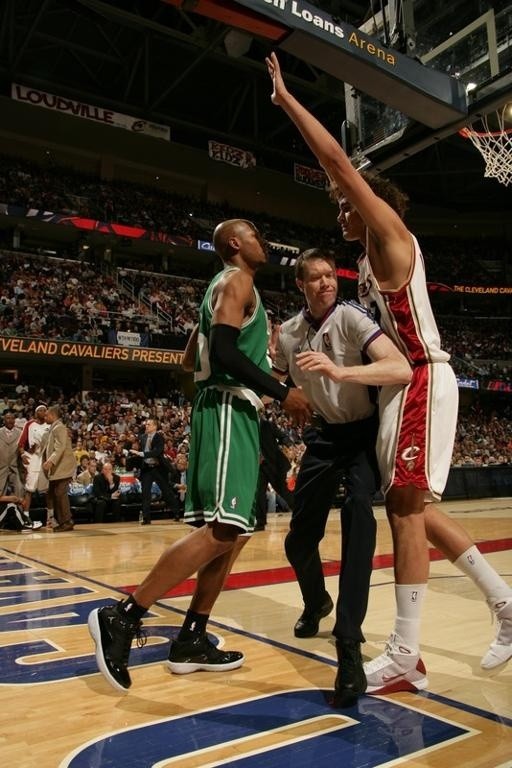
[260,246,412,702]
[88,216,315,692]
[264,49,512,694]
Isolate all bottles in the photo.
[139,509,144,524]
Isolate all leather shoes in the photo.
[335,637,367,698]
[294,591,333,637]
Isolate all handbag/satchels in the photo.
[0,502,25,530]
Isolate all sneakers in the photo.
[166,631,245,675]
[87,598,147,691]
[21,512,34,528]
[53,518,75,533]
[361,631,428,695]
[480,597,512,670]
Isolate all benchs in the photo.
[104,490,171,522]
[24,492,98,528]
